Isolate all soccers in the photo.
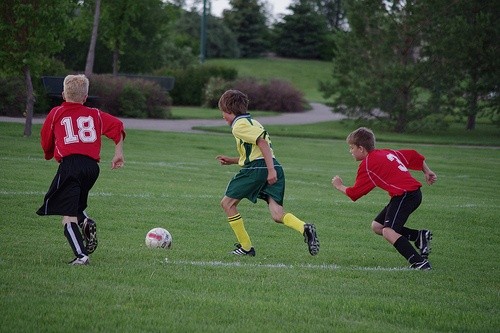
[145,228,173,251]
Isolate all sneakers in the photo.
[231,244,256,257]
[303,223,320,256]
[82,218,98,253]
[410,259,432,269]
[67,257,89,266]
[414,230,433,259]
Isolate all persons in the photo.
[36,74,126,264]
[215,90,320,255]
[331,127,437,270]
